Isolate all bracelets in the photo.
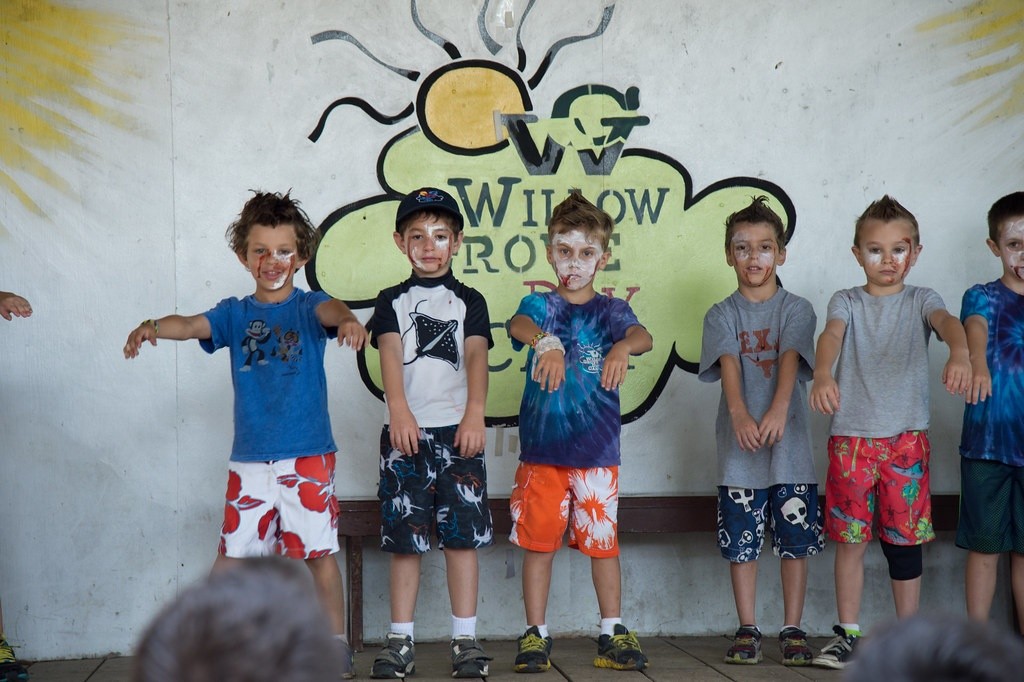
[141,319,159,337]
[529,331,549,349]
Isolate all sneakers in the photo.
[724,624,763,664]
[449,635,494,677]
[0,635,29,682]
[812,625,864,669]
[514,625,553,672]
[593,623,649,670]
[334,638,356,679]
[369,632,416,679]
[777,627,813,666]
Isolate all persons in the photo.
[506,188,655,672]
[841,601,1024,682]
[959,190,1024,638]
[0,291,33,682]
[697,195,818,668]
[370,186,496,680]
[808,195,972,669]
[122,187,370,679]
[127,554,348,682]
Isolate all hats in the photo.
[396,187,464,230]
